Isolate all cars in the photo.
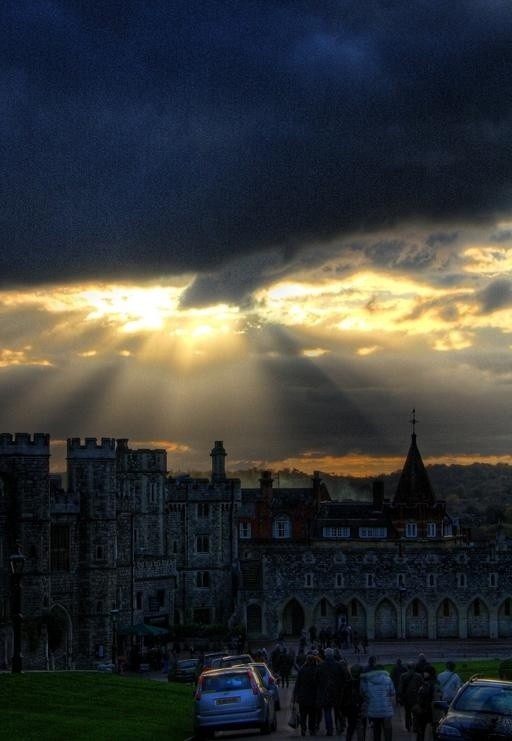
[196,652,281,739]
[169,657,200,680]
[139,663,151,672]
[97,661,116,670]
[432,674,512,740]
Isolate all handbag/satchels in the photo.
[287,712,301,729]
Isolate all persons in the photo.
[290,653,460,740]
[236,634,344,688]
[168,651,175,680]
[308,621,368,653]
